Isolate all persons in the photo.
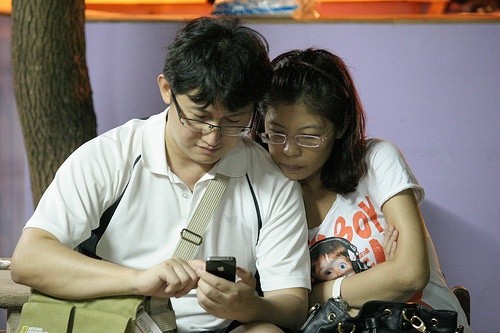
[246,45,475,333]
[6,10,314,333]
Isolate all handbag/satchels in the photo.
[301,298,464,333]
[17,288,151,333]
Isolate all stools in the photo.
[446,284,472,328]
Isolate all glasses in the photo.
[255,110,336,148]
[171,88,257,137]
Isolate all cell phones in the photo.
[203,256,238,286]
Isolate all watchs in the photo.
[331,275,350,313]
